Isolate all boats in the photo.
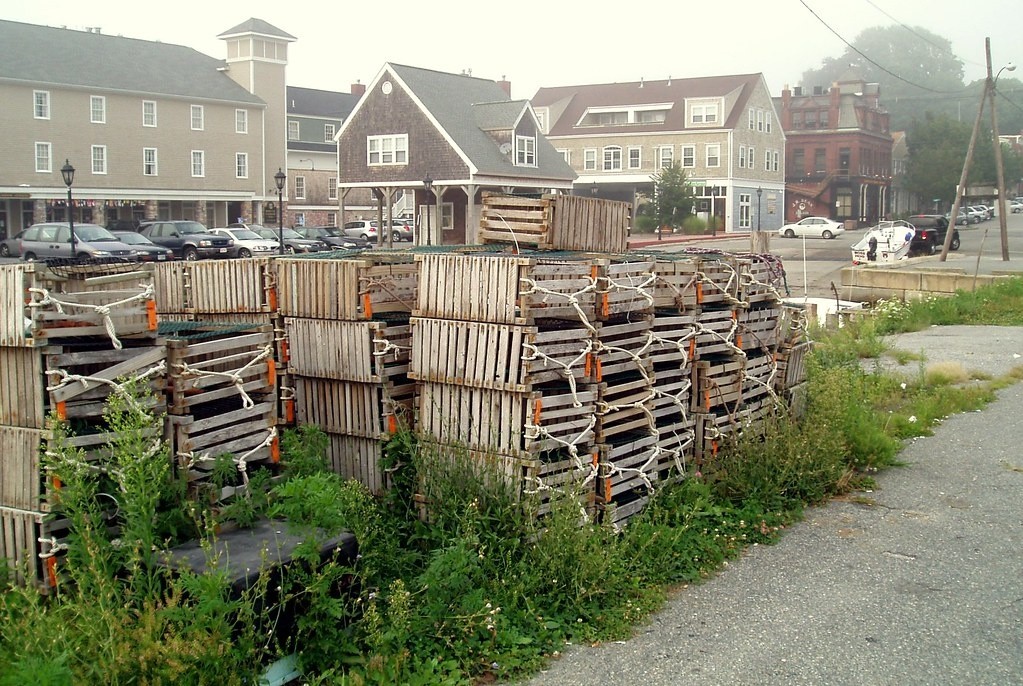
[852,218,916,263]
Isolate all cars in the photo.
[341,219,385,242]
[1009,201,1023,213]
[0,227,56,258]
[1014,197,1023,203]
[384,219,413,242]
[941,209,974,226]
[779,216,845,240]
[227,223,371,256]
[209,227,279,259]
[97,232,176,264]
[961,205,995,223]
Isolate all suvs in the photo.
[136,221,234,262]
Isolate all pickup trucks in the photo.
[904,215,961,256]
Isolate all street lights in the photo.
[422,170,433,245]
[712,185,717,235]
[274,167,288,255]
[58,160,78,263]
[589,181,598,197]
[757,186,763,232]
[989,62,1018,261]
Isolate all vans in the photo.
[18,222,138,264]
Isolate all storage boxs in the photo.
[0,195,817,534]
